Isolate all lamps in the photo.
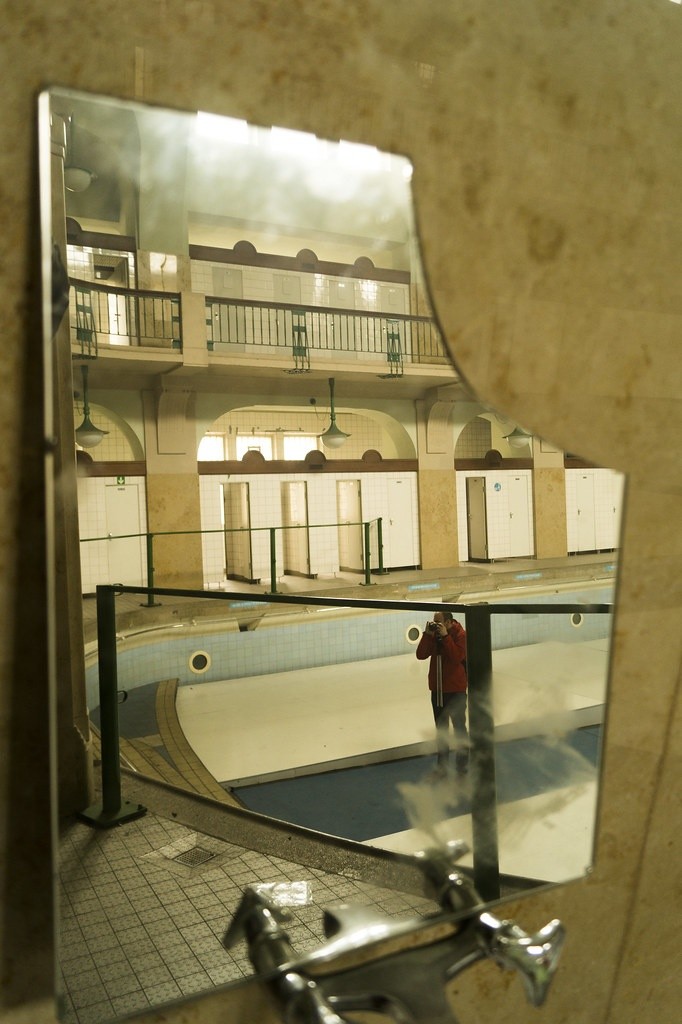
[501,424,534,449]
[315,378,352,449]
[74,364,110,449]
[64,167,93,193]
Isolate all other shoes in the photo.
[425,766,446,787]
[453,766,469,788]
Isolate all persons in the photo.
[415,611,467,777]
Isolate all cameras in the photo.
[429,622,441,631]
